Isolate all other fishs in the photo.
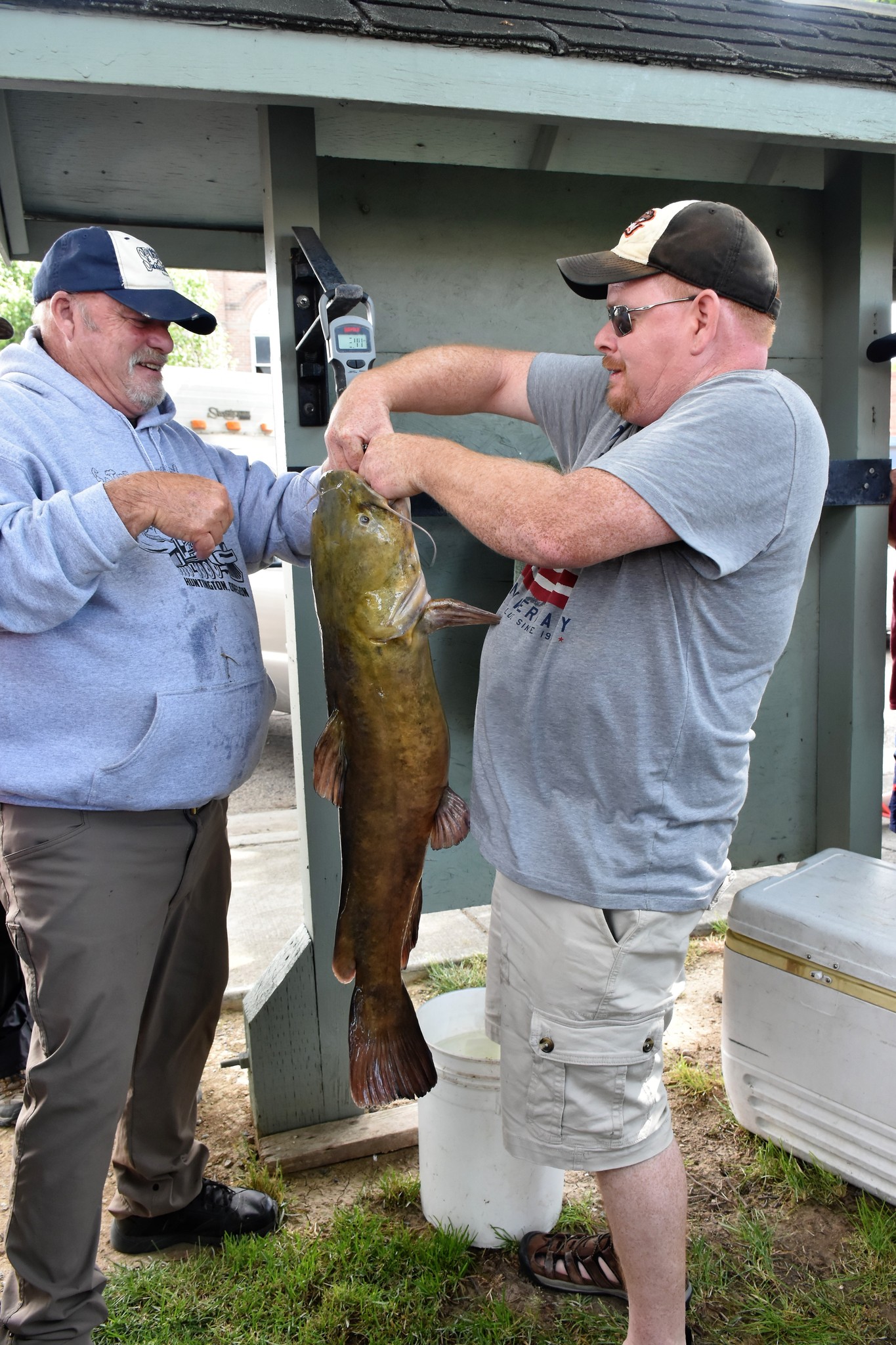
[300,468,502,1110]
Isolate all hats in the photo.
[33,225,217,337]
[553,200,783,320]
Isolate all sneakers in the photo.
[0,1070,27,1125]
[110,1178,279,1254]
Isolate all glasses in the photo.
[608,296,697,337]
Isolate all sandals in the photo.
[524,1234,693,1306]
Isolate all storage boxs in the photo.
[716,844,896,1207]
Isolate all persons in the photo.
[0,224,328,1345]
[323,197,830,1345]
